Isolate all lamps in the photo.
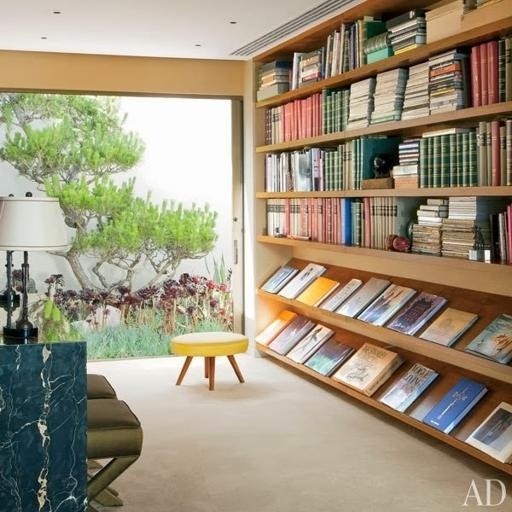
[1,192,69,340]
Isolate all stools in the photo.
[169,330,249,391]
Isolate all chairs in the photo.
[87,373,142,509]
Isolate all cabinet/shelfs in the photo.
[252,0,511,474]
